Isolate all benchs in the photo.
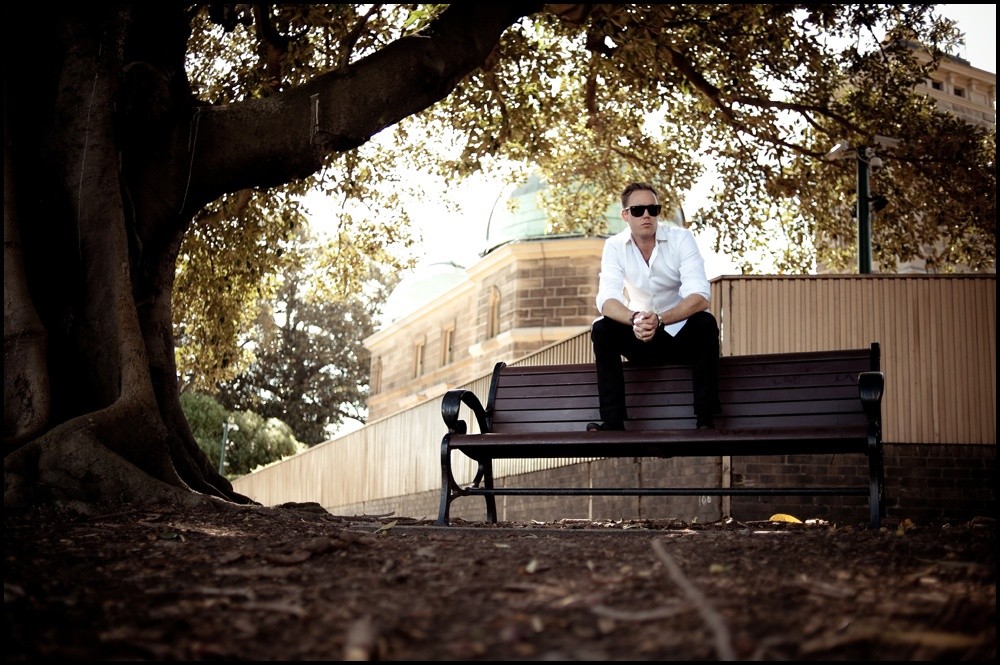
[433,343,886,527]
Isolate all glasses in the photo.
[625,204,662,217]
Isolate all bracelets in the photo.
[656,313,664,327]
[630,311,641,325]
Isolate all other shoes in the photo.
[586,422,625,432]
[696,417,716,429]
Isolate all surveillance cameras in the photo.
[872,133,906,154]
[823,138,849,162]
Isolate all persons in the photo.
[588,181,725,432]
